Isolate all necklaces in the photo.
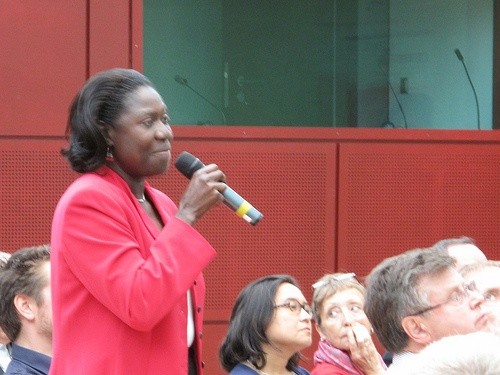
[136,194,146,202]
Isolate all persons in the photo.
[46,67,226,375]
[0,238,500,375]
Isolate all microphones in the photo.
[174,76,226,126]
[174,151,263,226]
[376,63,407,128]
[454,49,480,130]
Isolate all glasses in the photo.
[272,298,312,315]
[406,281,479,317]
